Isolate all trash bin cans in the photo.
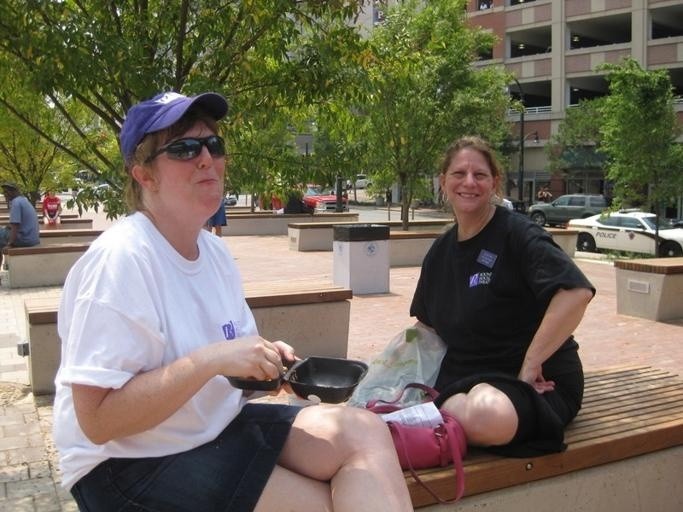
[284,190,304,214]
[332,224,390,295]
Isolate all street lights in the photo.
[501,71,526,214]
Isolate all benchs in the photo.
[215,206,363,239]
[25,278,352,398]
[612,256,683,322]
[286,215,457,258]
[0,198,103,290]
[388,226,581,264]
[404,362,683,512]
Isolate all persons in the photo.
[52,92,415,512]
[536,187,543,202]
[0,182,40,278]
[206,198,227,237]
[543,186,552,203]
[407,136,595,457]
[42,191,61,229]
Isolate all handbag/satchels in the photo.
[366,400,467,469]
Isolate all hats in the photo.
[119,90,229,166]
[2,182,18,190]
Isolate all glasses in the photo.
[142,135,225,163]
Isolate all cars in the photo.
[253,180,350,216]
[528,192,610,227]
[346,173,373,189]
[569,209,683,260]
[76,182,121,198]
